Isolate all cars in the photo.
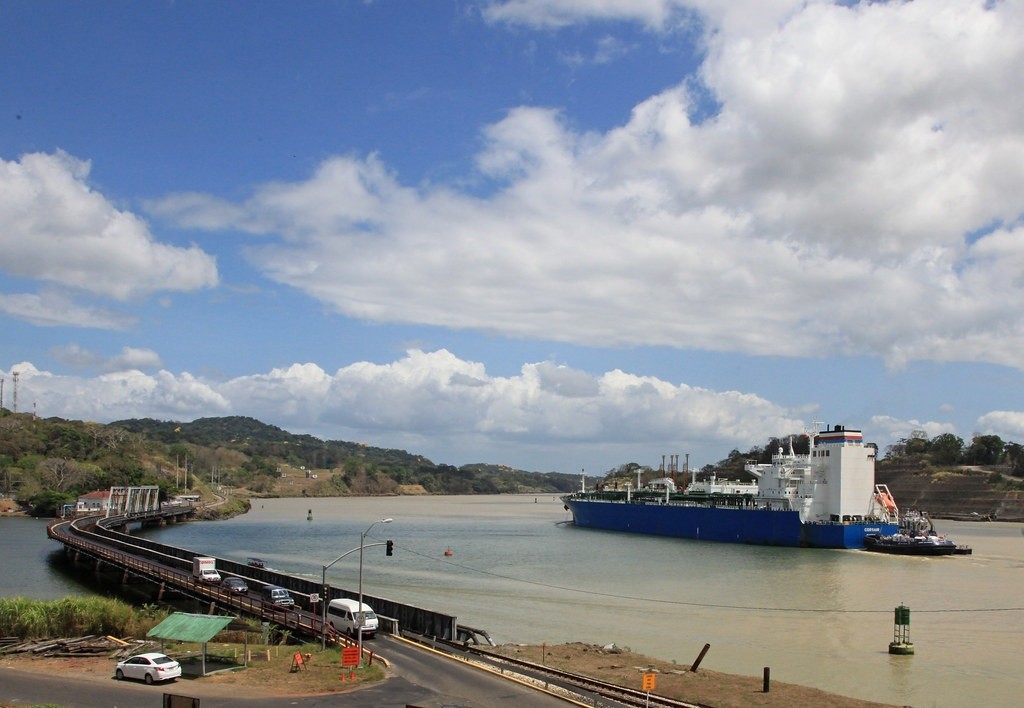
[223,577,248,596]
[115,651,182,684]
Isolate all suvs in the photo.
[262,584,295,610]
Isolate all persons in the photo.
[252,562,257,565]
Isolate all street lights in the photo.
[359,518,394,658]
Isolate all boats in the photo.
[864,509,972,556]
[560,424,900,549]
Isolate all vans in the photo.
[327,598,379,638]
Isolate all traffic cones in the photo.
[341,674,344,681]
[349,672,354,680]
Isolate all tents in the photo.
[146,611,263,674]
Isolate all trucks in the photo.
[193,557,221,585]
